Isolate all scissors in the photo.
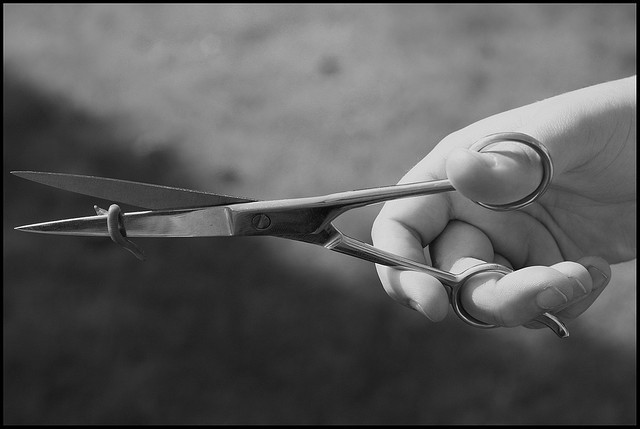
[11,133,570,339]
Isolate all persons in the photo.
[369,73,638,329]
